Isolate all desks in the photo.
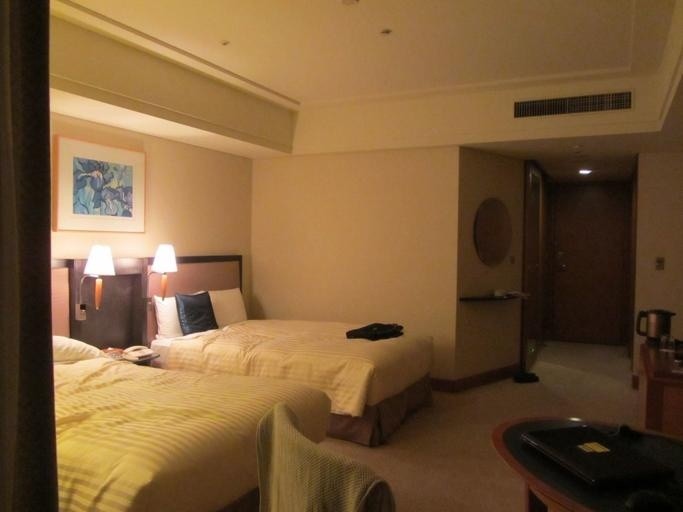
[639,340,682,435]
[490,412,681,512]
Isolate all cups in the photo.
[655,347,675,377]
[658,334,676,353]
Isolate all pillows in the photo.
[153,296,184,340]
[207,287,248,329]
[174,290,219,337]
[52,335,106,364]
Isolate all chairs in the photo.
[269,402,363,512]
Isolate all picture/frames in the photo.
[50,133,149,234]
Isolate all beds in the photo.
[148,287,435,448]
[52,334,334,512]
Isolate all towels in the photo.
[346,322,405,341]
[253,400,394,512]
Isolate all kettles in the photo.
[636,309,676,343]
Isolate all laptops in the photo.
[523,424,653,486]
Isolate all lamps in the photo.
[150,243,179,301]
[74,244,116,322]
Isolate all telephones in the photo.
[122,344,154,360]
